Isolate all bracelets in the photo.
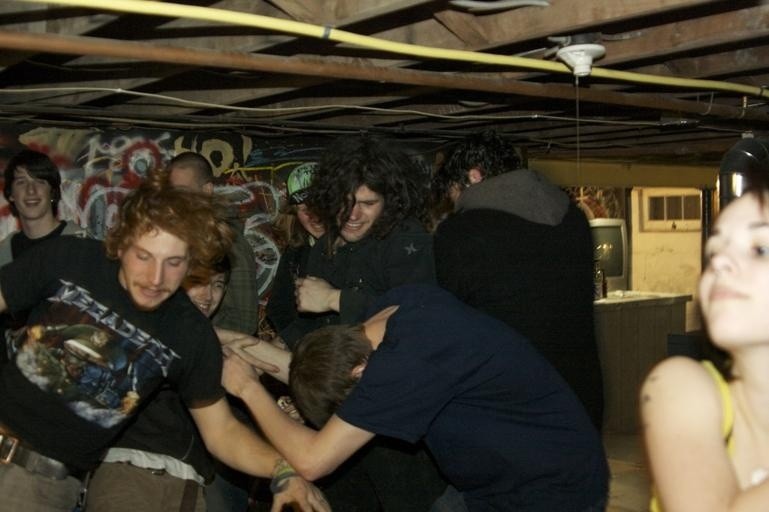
[267,462,300,487]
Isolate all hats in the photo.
[286,162,318,205]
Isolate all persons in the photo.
[85,249,307,510]
[1,150,103,337]
[166,152,259,509]
[266,161,329,354]
[220,281,607,511]
[640,182,768,511]
[427,134,605,509]
[0,168,335,511]
[265,136,438,510]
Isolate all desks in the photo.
[592,286,695,437]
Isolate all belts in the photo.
[0,431,68,481]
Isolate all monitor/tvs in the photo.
[588,218,628,293]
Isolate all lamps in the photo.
[556,40,611,79]
